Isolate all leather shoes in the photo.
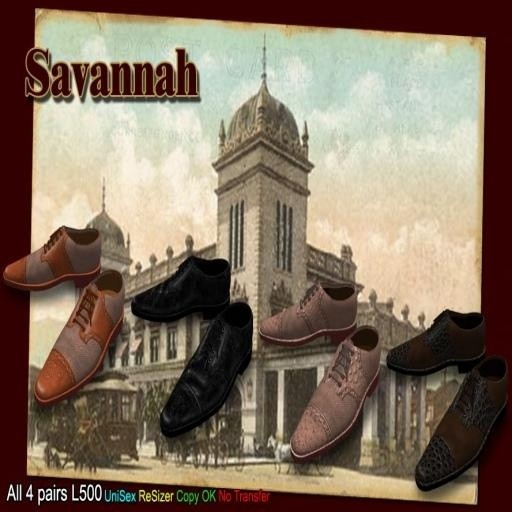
[33,269,125,408]
[413,355,509,491]
[130,255,233,323]
[258,280,358,347]
[2,225,103,292]
[159,301,254,438]
[385,310,487,376]
[290,324,382,464]
